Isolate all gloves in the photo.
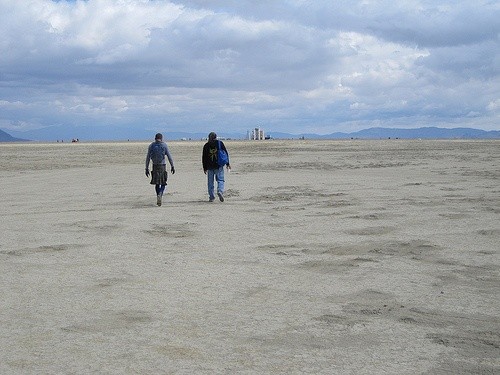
[146,169,150,177]
[171,167,175,174]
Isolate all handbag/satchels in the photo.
[217,140,228,166]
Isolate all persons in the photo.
[145,133,175,207]
[202,132,231,202]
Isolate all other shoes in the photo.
[209,197,215,202]
[157,194,161,206]
[217,192,224,202]
